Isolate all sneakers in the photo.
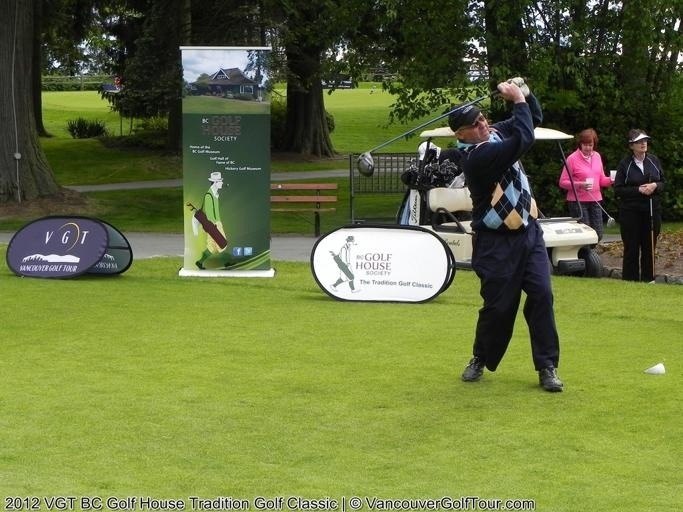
[539,367,564,392]
[461,356,484,382]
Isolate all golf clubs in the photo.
[574,175,616,229]
[357,82,525,177]
[649,194,656,284]
[186,203,197,212]
[329,251,337,256]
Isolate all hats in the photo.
[627,128,651,142]
[418,141,432,161]
[448,102,481,133]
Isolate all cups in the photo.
[610,170,617,182]
[587,178,593,189]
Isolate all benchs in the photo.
[270,184,338,236]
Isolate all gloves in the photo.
[507,76,531,98]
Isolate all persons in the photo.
[615,129,665,282]
[330,236,361,294]
[195,172,236,269]
[449,77,565,392]
[559,129,616,249]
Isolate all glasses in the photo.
[629,137,647,144]
